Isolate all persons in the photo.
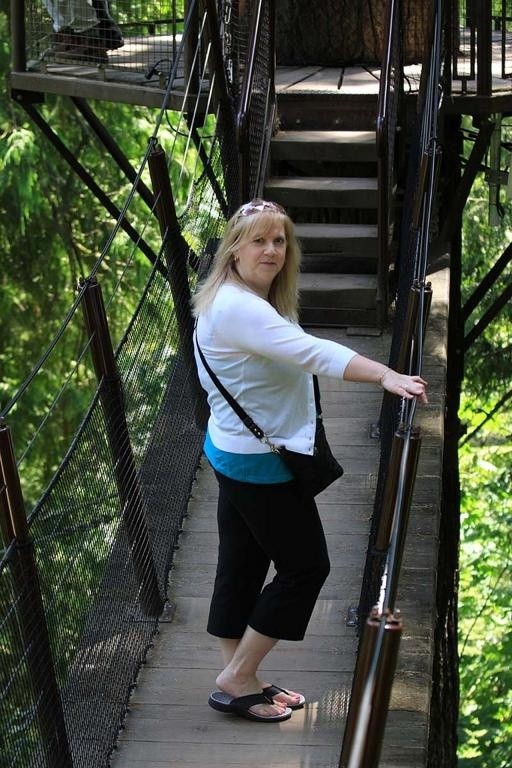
[187,197,430,722]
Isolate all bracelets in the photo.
[379,366,392,385]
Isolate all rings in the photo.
[404,382,409,390]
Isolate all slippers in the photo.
[263,684,306,708]
[208,692,292,722]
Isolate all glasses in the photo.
[238,201,287,216]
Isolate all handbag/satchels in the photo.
[275,418,344,498]
